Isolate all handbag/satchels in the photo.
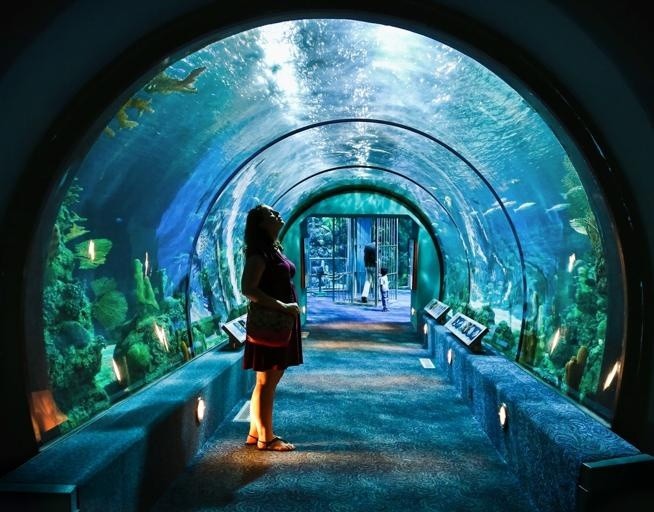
[246,307,297,347]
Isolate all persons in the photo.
[360,240,379,302]
[241,205,302,452]
[378,268,389,312]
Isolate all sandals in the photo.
[244,435,296,451]
